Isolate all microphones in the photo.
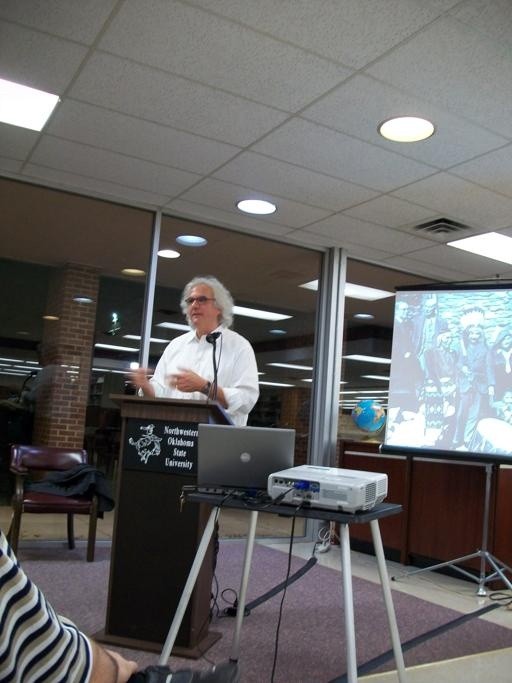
[206,332,221,343]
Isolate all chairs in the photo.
[86,404,122,480]
[8,444,99,561]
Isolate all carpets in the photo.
[0,504,304,539]
[15,539,512,683]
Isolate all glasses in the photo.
[185,295,215,306]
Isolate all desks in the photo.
[158,494,403,683]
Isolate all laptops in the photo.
[196,423,296,488]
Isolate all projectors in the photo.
[267,464,388,515]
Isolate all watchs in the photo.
[202,381,211,393]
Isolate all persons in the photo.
[0,529,239,683]
[385,292,512,456]
[125,277,260,623]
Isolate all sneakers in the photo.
[142,656,237,683]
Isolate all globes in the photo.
[352,400,387,443]
